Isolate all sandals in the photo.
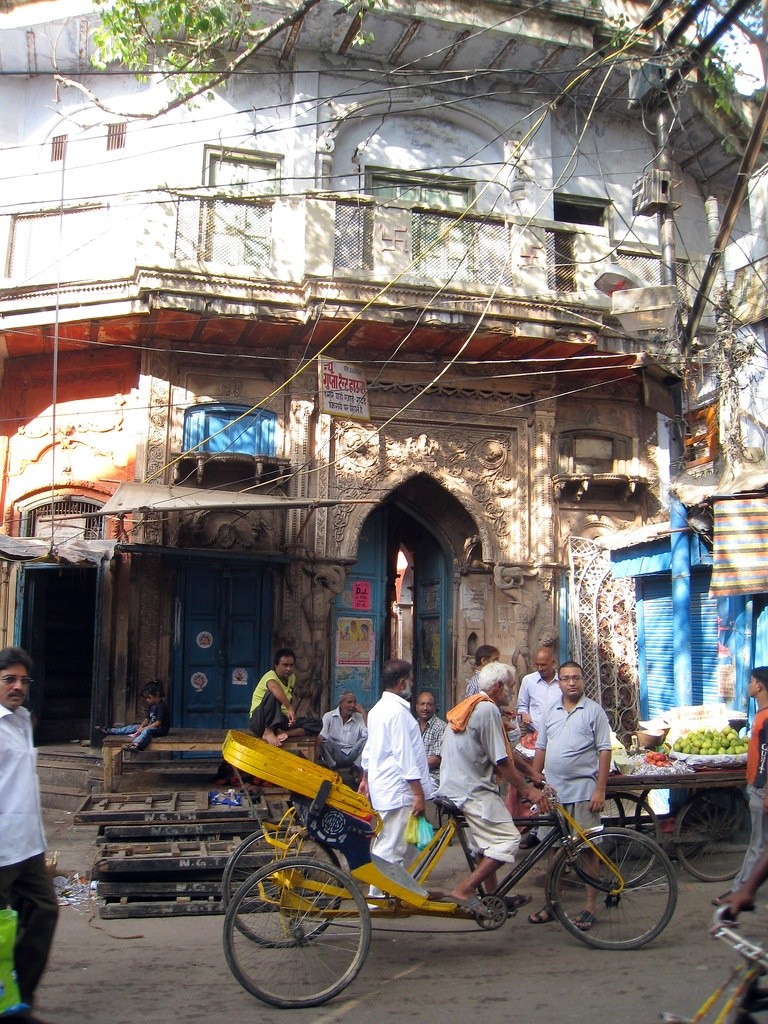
[95,724,110,736]
[123,743,139,754]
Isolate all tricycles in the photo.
[217,729,751,1009]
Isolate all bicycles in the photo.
[661,900,768,1024]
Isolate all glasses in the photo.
[559,676,584,683]
[0,676,35,685]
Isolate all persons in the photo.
[0,647,58,1023]
[511,625,561,666]
[248,649,612,931]
[711,665,768,907]
[95,680,170,750]
[724,849,767,917]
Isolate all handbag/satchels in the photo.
[0,908,32,1018]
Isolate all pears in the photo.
[672,726,750,755]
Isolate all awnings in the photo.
[0,535,116,564]
[40,478,378,523]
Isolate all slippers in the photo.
[712,890,755,913]
[520,831,538,849]
[572,909,594,931]
[504,893,533,913]
[448,893,493,920]
[529,904,553,923]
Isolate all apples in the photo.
[646,751,673,767]
